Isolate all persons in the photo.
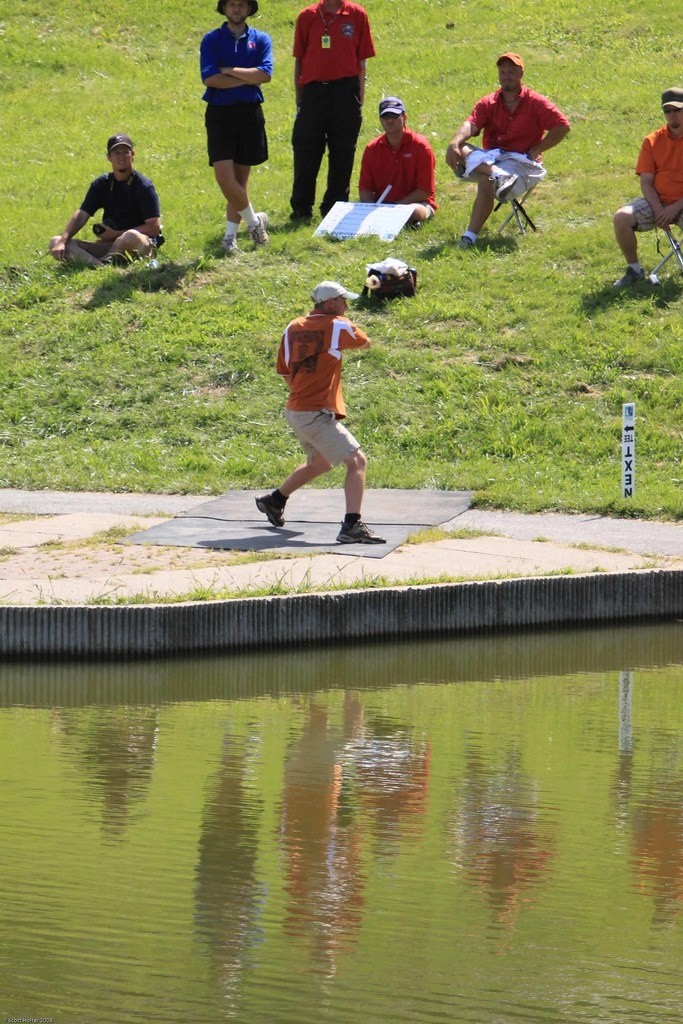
[255,280,386,545]
[323,98,437,243]
[612,87,683,289]
[49,134,165,269]
[284,0,376,230]
[200,0,274,257]
[446,53,571,253]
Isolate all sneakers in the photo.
[251,212,269,247]
[489,174,518,199]
[255,493,284,527]
[457,236,473,249]
[335,518,386,544]
[220,237,245,255]
[613,267,645,286]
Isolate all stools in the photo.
[647,227,683,276]
[498,185,536,238]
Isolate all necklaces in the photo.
[505,98,517,106]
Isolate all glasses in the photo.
[663,107,681,113]
[382,113,399,119]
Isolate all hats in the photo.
[107,136,132,152]
[496,52,524,75]
[217,0,258,16]
[312,281,359,305]
[660,87,683,108]
[379,97,405,116]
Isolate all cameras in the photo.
[93,218,118,236]
